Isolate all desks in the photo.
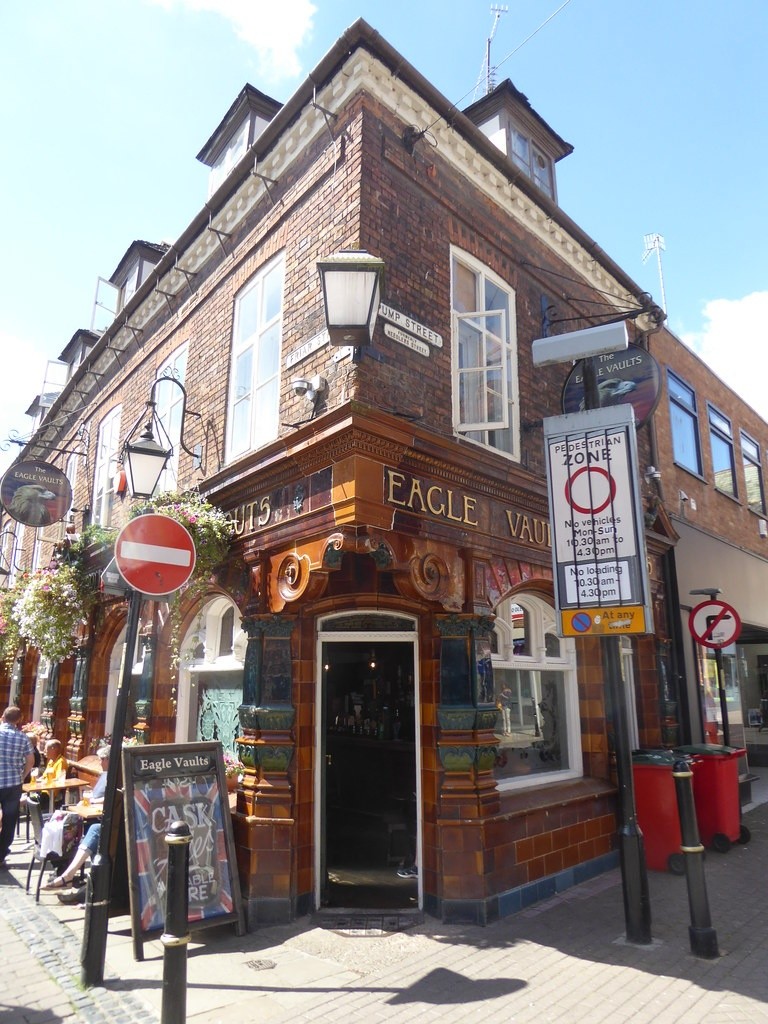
[21,778,90,814]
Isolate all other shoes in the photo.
[397,865,418,879]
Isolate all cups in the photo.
[82,790,91,806]
[30,767,38,786]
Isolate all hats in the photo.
[96,745,111,758]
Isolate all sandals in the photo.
[38,876,73,890]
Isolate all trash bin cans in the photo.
[630,749,688,874]
[672,743,751,852]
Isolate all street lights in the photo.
[688,587,731,748]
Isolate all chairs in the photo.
[19,767,101,906]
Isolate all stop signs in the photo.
[113,514,196,596]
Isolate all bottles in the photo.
[56,769,66,786]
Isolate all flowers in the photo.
[0,521,120,671]
[126,488,250,712]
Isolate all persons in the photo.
[498,682,512,737]
[39,823,101,890]
[20,739,68,805]
[46,744,111,881]
[0,706,35,868]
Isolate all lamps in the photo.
[123,376,210,500]
[315,248,386,348]
